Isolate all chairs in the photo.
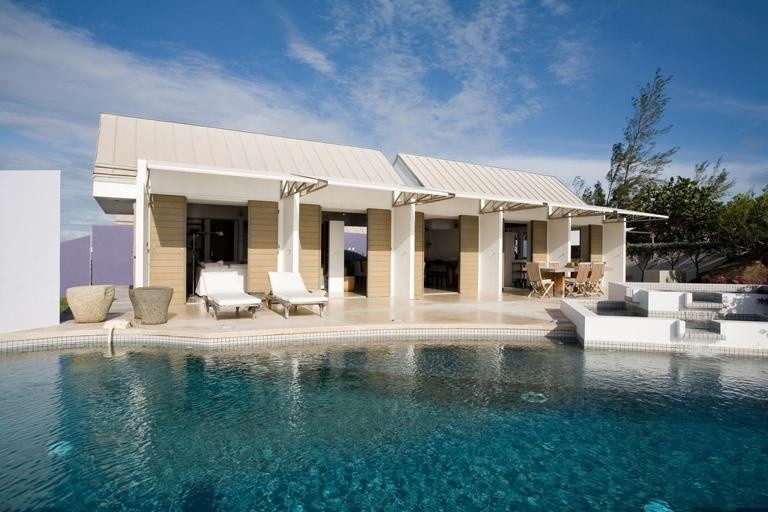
[266,271,329,319]
[361,261,367,292]
[512,260,614,301]
[201,271,262,320]
[353,260,364,289]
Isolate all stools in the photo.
[344,275,356,292]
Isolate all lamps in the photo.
[425,221,431,231]
[425,239,433,261]
[189,231,225,298]
[454,220,459,229]
